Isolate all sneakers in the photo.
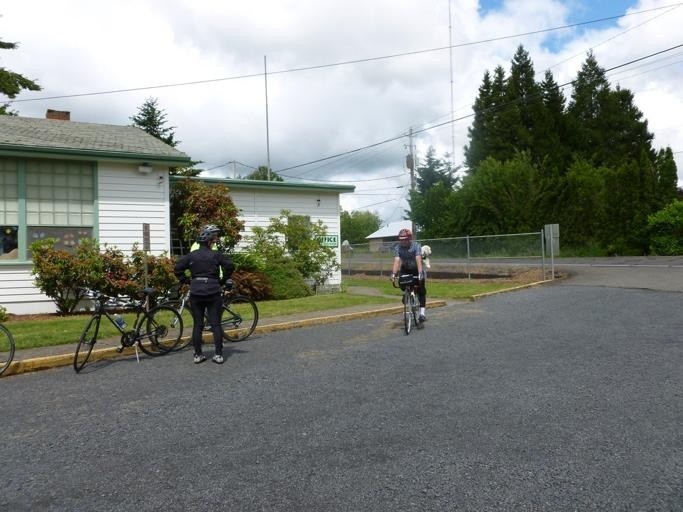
[213,355,224,363]
[418,316,426,322]
[194,354,205,363]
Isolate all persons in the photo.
[390,229,427,322]
[173,224,235,365]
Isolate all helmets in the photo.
[196,224,221,244]
[399,229,412,240]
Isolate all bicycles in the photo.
[391,272,425,335]
[72,278,258,374]
[0,322,15,379]
